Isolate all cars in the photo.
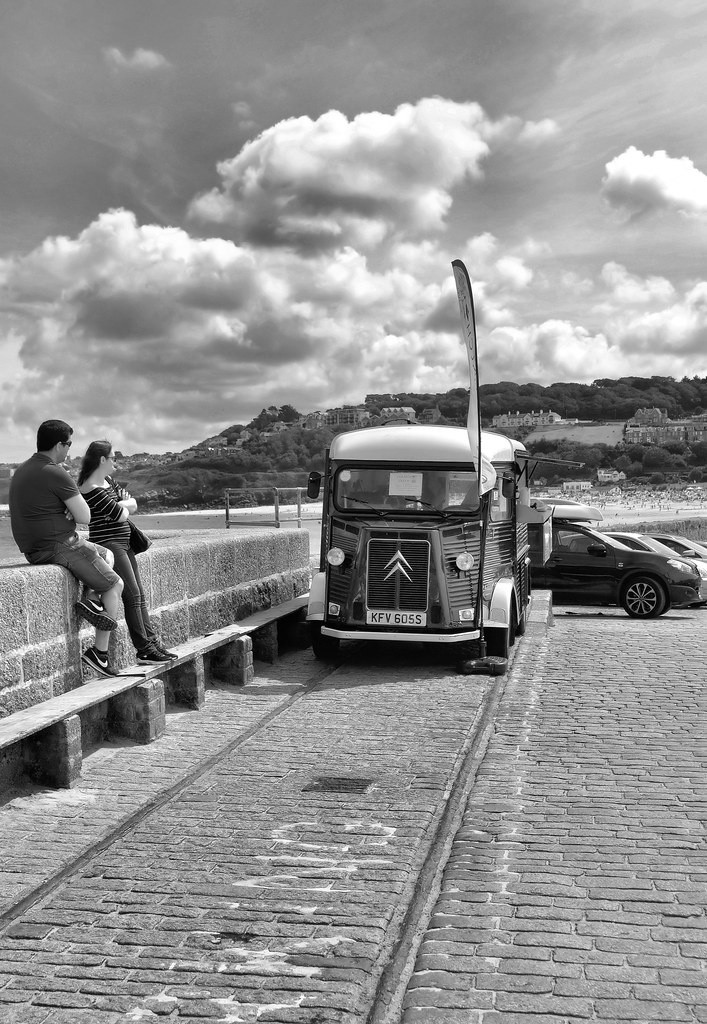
[641,532,707,563]
[560,530,707,610]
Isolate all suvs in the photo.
[528,521,703,620]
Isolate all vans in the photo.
[304,421,586,664]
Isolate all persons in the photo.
[9,419,124,678]
[529,481,707,517]
[76,440,178,664]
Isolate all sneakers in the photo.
[74,598,118,631]
[81,647,117,677]
[157,647,178,660]
[136,650,172,664]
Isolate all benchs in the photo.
[0,593,309,788]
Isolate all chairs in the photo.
[385,495,417,509]
[461,480,478,508]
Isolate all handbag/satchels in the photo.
[105,475,153,555]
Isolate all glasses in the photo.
[54,441,72,447]
[103,455,116,462]
